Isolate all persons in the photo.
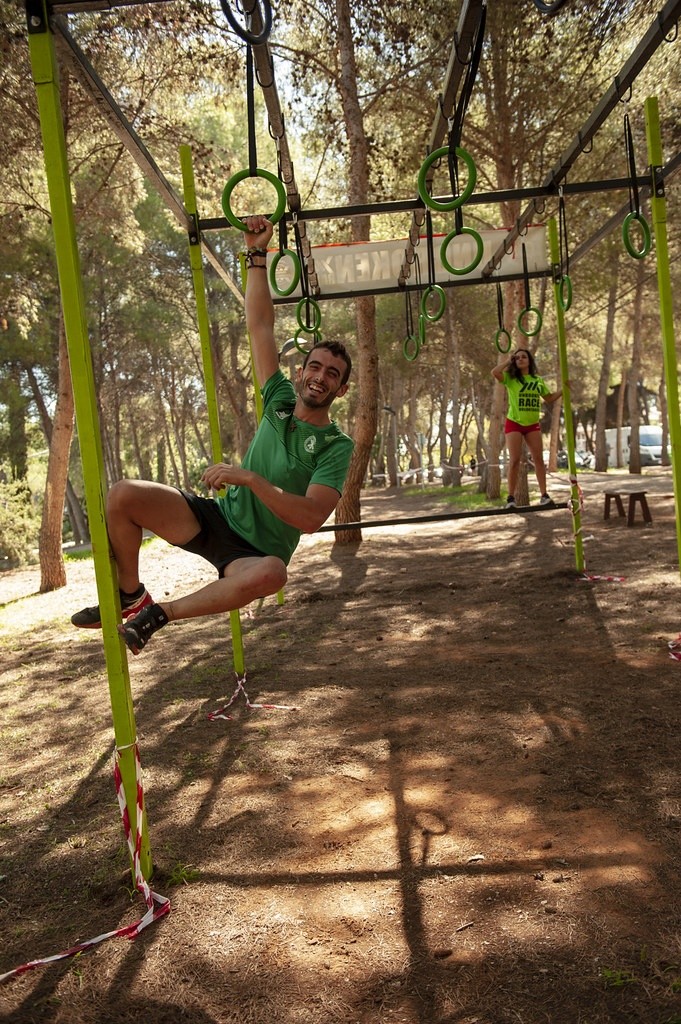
[491,350,572,510]
[72,216,354,655]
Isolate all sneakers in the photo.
[505,494,517,509]
[540,493,555,505]
[71,583,156,629]
[116,603,169,655]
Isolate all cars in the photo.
[467,450,596,476]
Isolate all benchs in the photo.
[603,488,652,527]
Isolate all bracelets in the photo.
[242,250,267,270]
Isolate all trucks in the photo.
[606,425,671,468]
